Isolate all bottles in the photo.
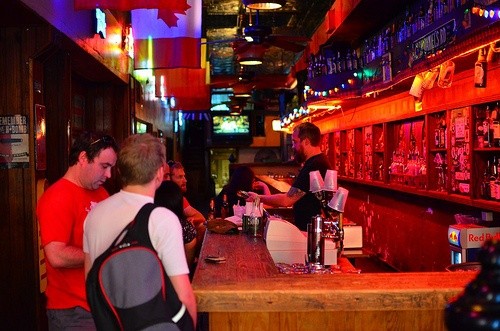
[379,162,384,181]
[248,203,264,236]
[474,106,500,201]
[207,199,215,221]
[434,119,447,191]
[122,24,134,58]
[221,195,229,218]
[474,49,487,88]
[307,0,467,80]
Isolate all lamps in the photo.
[237,41,264,65]
[244,0,286,10]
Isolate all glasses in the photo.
[90,135,118,150]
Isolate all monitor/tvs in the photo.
[212,110,254,139]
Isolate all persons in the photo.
[443,233,500,331]
[83,133,196,331]
[236,122,331,231]
[213,164,271,219]
[154,161,205,278]
[37,129,119,331]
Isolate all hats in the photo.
[207,219,239,234]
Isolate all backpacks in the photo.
[86,203,193,331]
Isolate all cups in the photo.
[409,73,423,98]
[309,169,349,213]
[423,72,439,90]
[437,60,455,89]
